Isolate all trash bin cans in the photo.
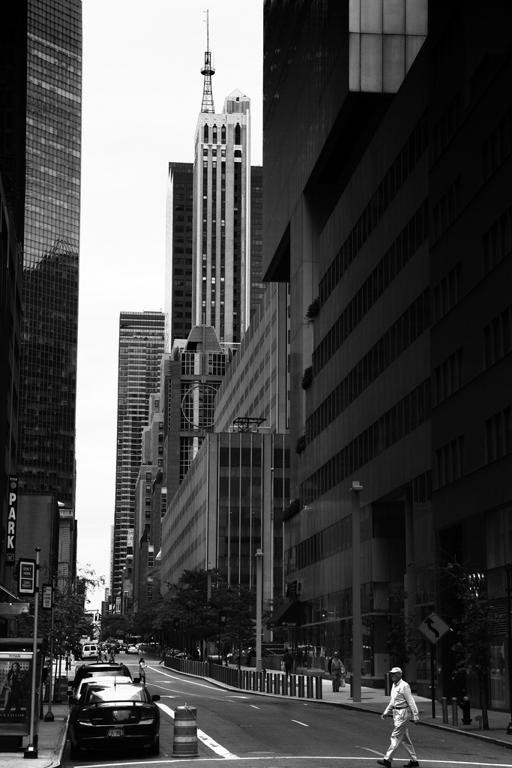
[385,673,394,696]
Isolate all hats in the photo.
[388,667,402,675]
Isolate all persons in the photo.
[159,647,166,664]
[0,662,27,713]
[283,649,294,679]
[96,646,115,664]
[329,649,346,692]
[136,657,149,687]
[374,666,422,768]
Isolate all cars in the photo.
[82,639,159,658]
[67,659,161,756]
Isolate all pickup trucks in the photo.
[174,646,223,666]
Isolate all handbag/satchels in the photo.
[340,673,345,687]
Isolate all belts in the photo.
[392,706,406,709]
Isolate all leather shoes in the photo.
[402,759,419,768]
[376,758,392,768]
